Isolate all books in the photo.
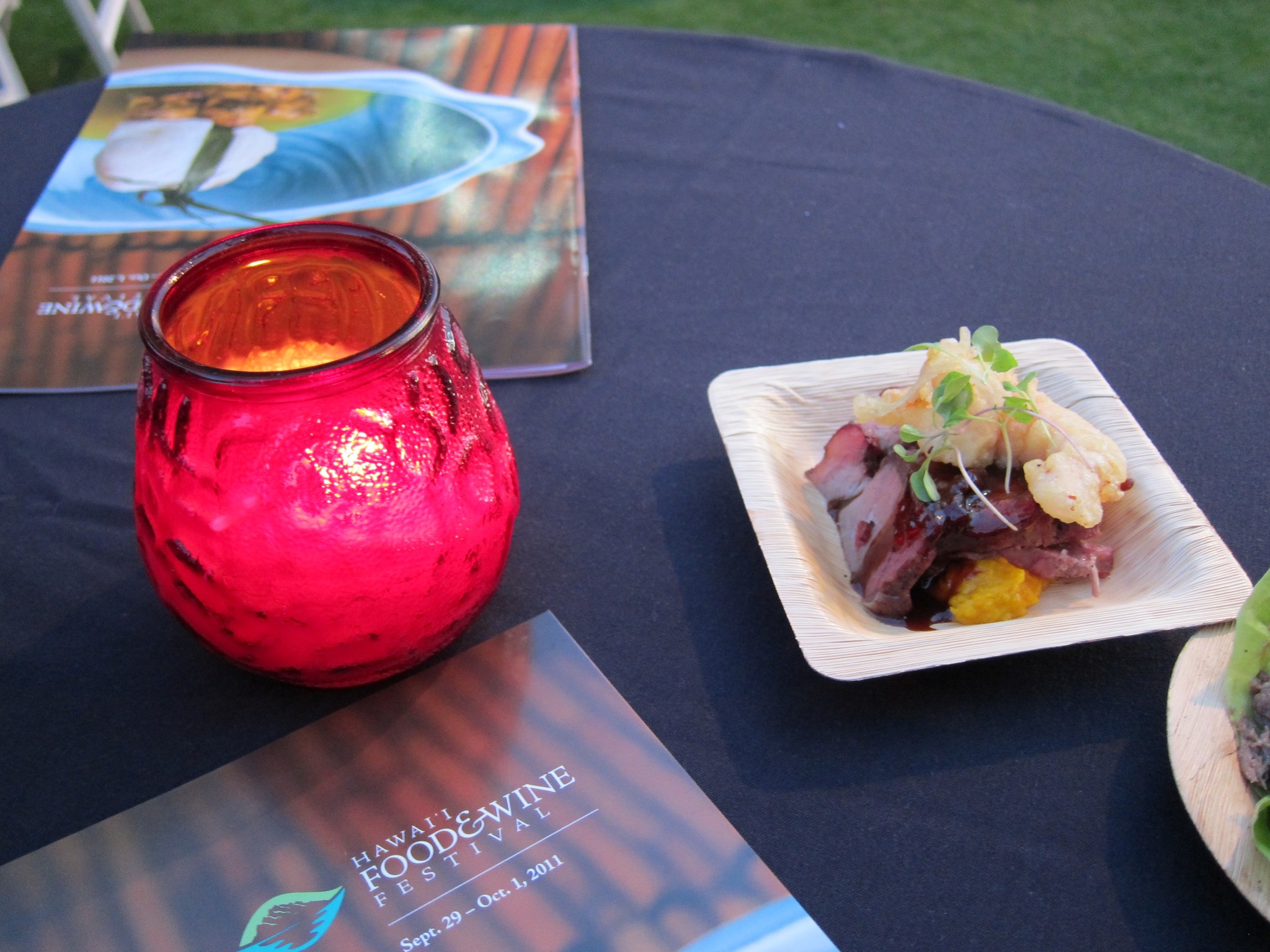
[0,609,847,951]
[1,20,595,395]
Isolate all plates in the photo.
[1167,619,1268,924]
[705,336,1252,681]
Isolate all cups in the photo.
[133,225,522,690]
